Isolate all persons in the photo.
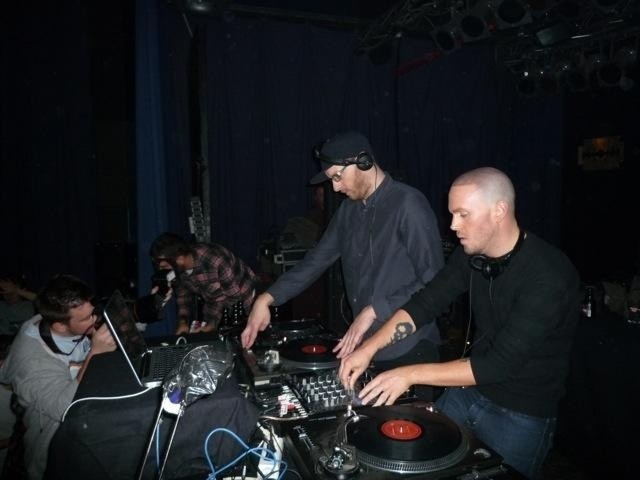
[243,128,445,402]
[338,167,583,479]
[0,274,119,480]
[167,230,258,333]
[135,234,178,337]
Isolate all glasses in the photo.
[328,166,346,183]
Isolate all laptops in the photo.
[104,287,199,389]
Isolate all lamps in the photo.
[430,24,459,57]
[493,1,530,30]
[460,12,488,43]
[595,58,625,88]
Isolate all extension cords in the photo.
[257,436,284,479]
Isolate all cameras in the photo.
[90,293,165,330]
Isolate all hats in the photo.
[309,132,368,185]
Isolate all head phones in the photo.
[315,141,373,171]
[470,230,524,280]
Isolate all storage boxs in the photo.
[273,248,329,319]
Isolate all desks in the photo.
[44,327,527,480]
